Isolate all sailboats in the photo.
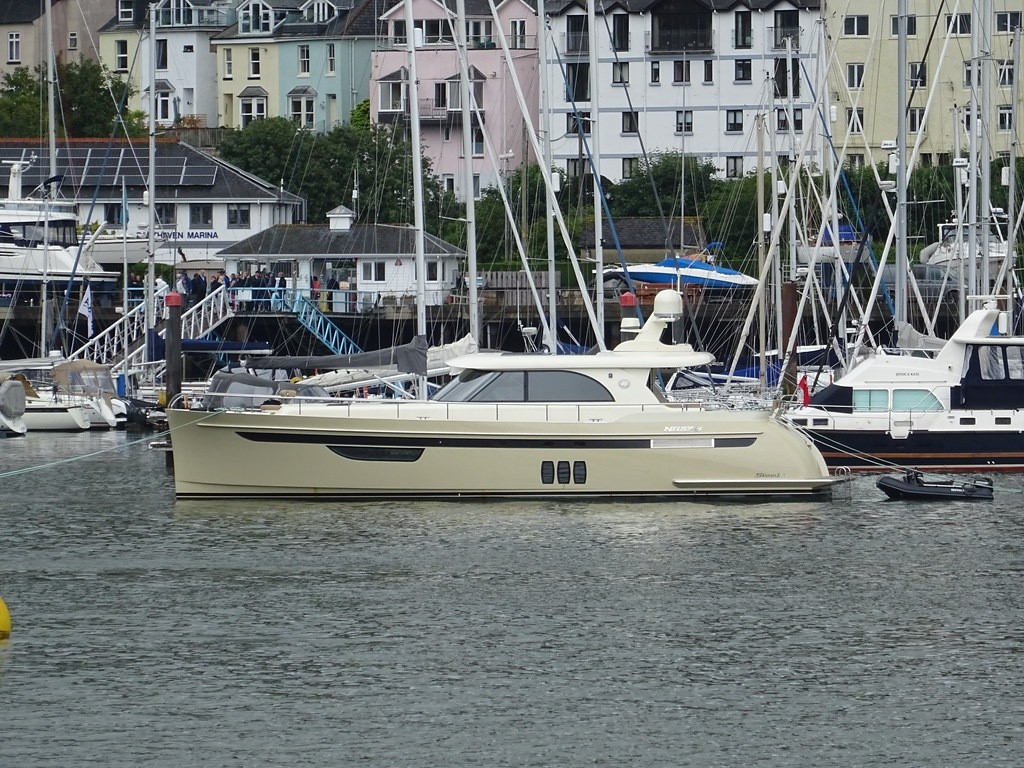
[0,0,1024,500]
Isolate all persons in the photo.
[326,274,340,312]
[456,273,466,303]
[210,271,286,312]
[311,276,321,310]
[129,274,166,314]
[176,270,207,314]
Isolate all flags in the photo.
[78,281,94,338]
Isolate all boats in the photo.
[876,468,995,501]
